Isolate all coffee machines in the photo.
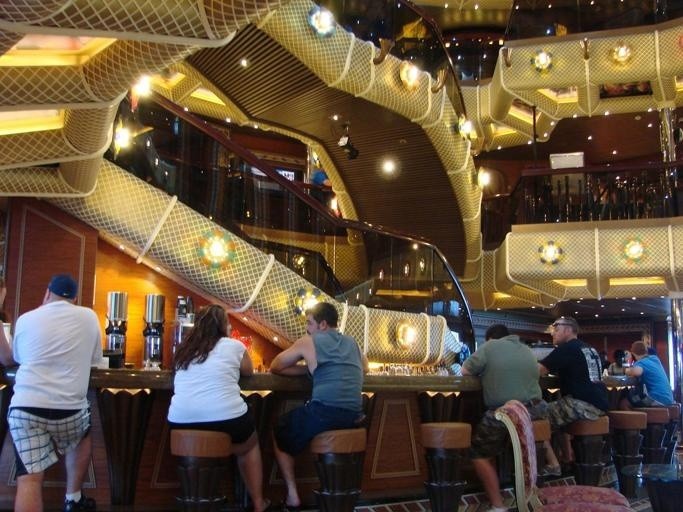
[143,294,165,368]
[105,292,126,361]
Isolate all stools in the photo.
[610,410,647,494]
[530,420,551,485]
[170,428,231,512]
[311,428,367,512]
[420,423,471,512]
[571,415,609,486]
[668,403,681,464]
[635,408,669,464]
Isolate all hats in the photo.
[47,274,80,299]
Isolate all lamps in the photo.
[473,164,489,187]
[530,49,553,73]
[307,5,336,39]
[621,238,647,261]
[394,319,413,349]
[198,229,234,270]
[609,42,635,63]
[538,240,563,266]
[457,112,473,141]
[293,286,327,317]
[399,58,419,93]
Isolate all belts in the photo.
[486,396,541,411]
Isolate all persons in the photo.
[0,279,18,369]
[537,317,609,479]
[7,275,103,512]
[165,304,272,512]
[461,324,548,512]
[309,165,332,206]
[269,302,370,512]
[597,341,673,412]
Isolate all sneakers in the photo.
[61,494,96,511]
[537,462,562,477]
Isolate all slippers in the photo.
[254,497,272,512]
[279,496,300,511]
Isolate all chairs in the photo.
[495,397,636,512]
